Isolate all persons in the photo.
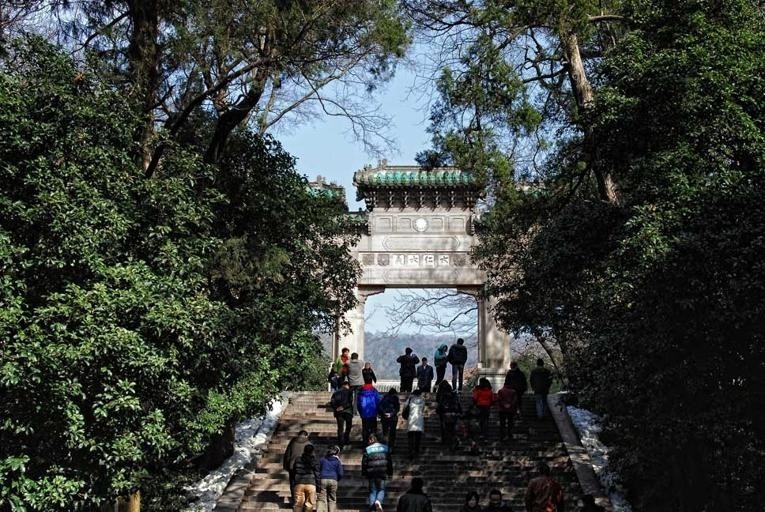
[284,430,343,511]
[361,433,394,511]
[496,379,520,445]
[461,488,509,511]
[327,347,377,415]
[472,378,495,430]
[330,381,428,460]
[504,361,528,421]
[466,404,481,451]
[530,358,553,419]
[575,494,606,512]
[397,477,432,511]
[435,379,463,443]
[396,339,468,392]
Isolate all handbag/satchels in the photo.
[402,405,409,420]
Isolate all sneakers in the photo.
[375,501,382,512]
